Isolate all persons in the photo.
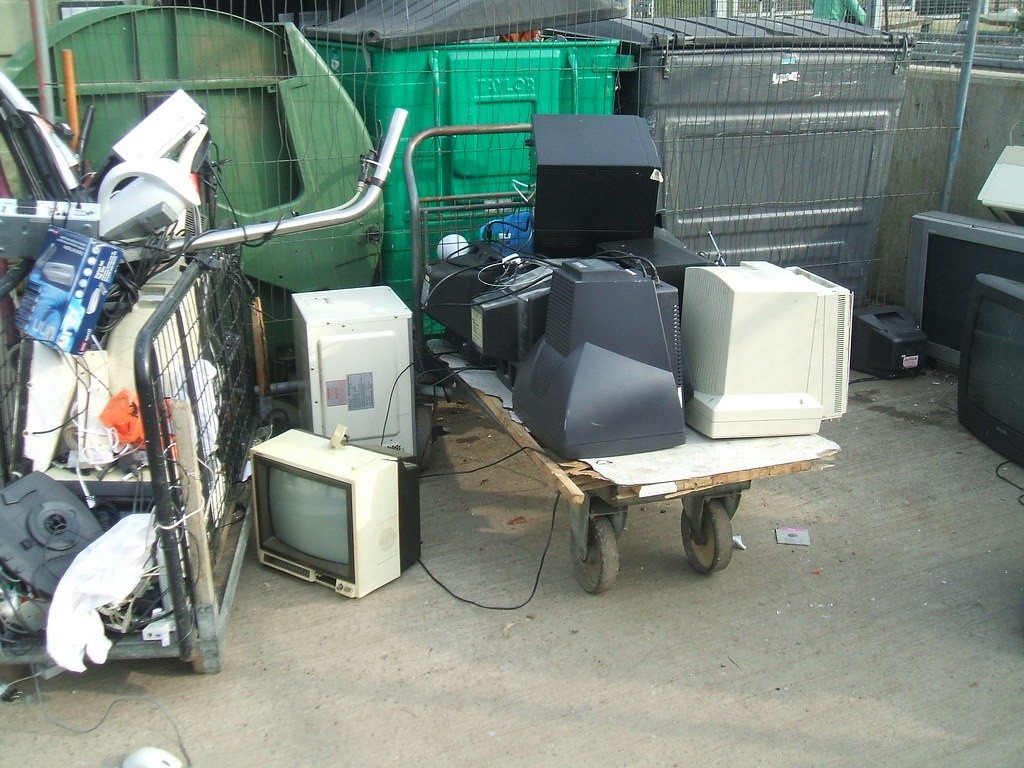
[810,0,867,26]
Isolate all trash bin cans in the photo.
[305,0,637,368]
[2,4,386,378]
[570,12,914,299]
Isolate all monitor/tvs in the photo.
[249,428,421,600]
[852,143,1024,474]
[416,222,852,464]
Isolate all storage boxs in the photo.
[15,223,124,352]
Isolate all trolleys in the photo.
[398,123,840,596]
